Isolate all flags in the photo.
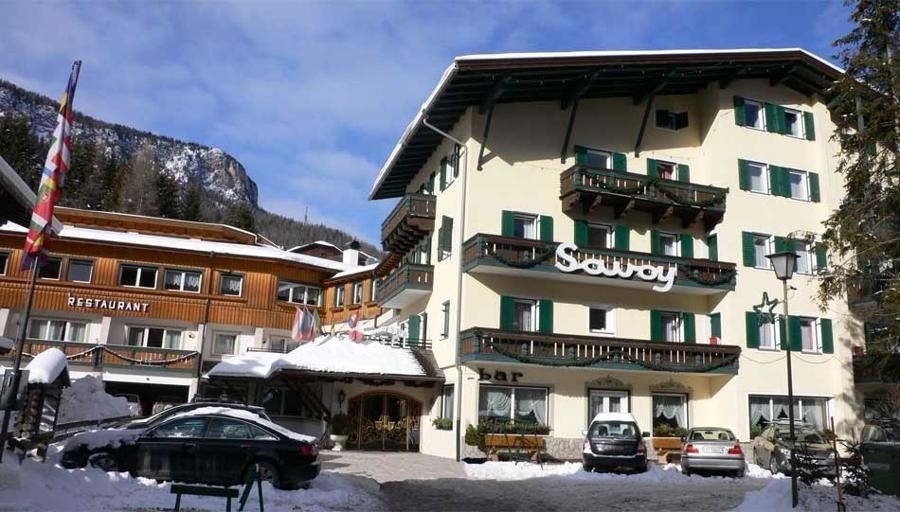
[347,309,360,330]
[312,309,321,338]
[290,307,304,344]
[301,305,315,341]
[19,58,82,271]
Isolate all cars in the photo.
[56,404,323,492]
[126,400,273,429]
[751,415,843,479]
[676,424,749,476]
[861,414,899,487]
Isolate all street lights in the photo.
[761,248,803,508]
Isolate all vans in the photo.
[580,410,650,474]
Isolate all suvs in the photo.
[149,391,183,416]
[114,390,144,418]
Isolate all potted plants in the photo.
[650,423,687,451]
[330,412,349,452]
[466,422,554,452]
